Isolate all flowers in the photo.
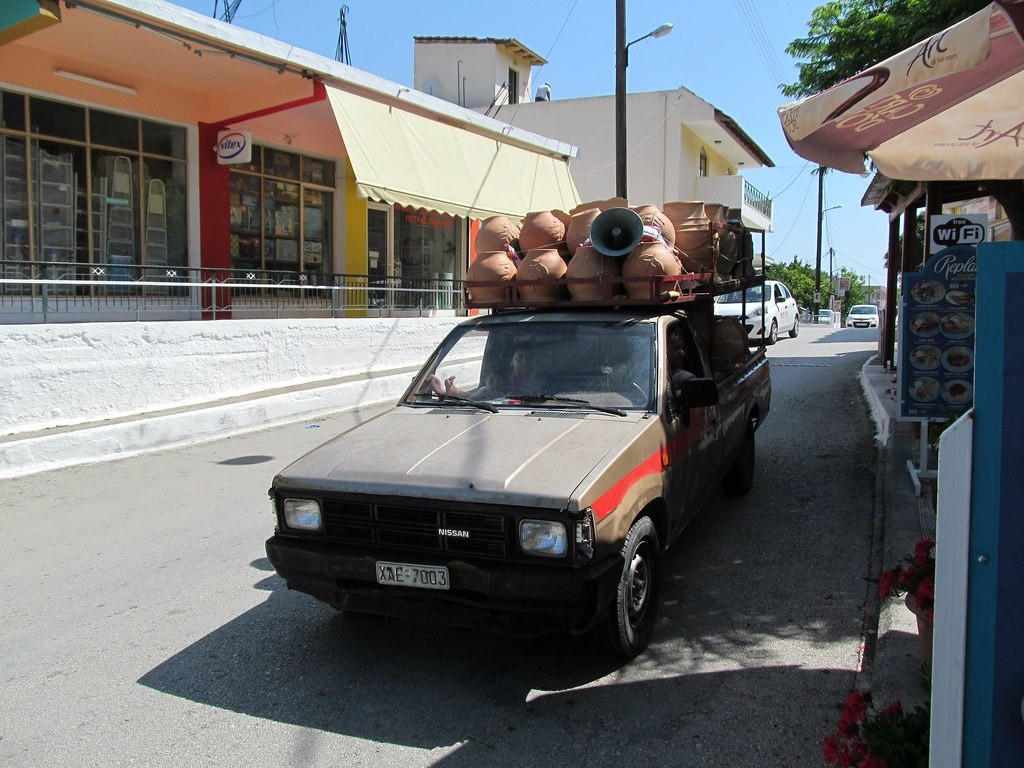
[878,540,941,619]
[817,687,931,768]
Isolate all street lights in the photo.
[813,204,842,322]
[617,23,673,201]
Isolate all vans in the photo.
[846,304,879,329]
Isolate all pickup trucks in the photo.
[266,306,771,660]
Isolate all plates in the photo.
[940,379,972,405]
[908,376,941,402]
[945,291,966,305]
[909,345,942,370]
[939,312,974,339]
[941,346,974,372]
[909,312,940,337]
[911,279,945,304]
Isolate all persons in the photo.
[427,329,699,411]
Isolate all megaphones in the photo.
[589,207,644,257]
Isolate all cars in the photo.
[714,280,800,345]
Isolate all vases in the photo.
[904,591,935,683]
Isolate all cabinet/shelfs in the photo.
[396,210,436,306]
[0,119,177,293]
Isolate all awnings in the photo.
[323,84,585,228]
[777,1,1024,183]
